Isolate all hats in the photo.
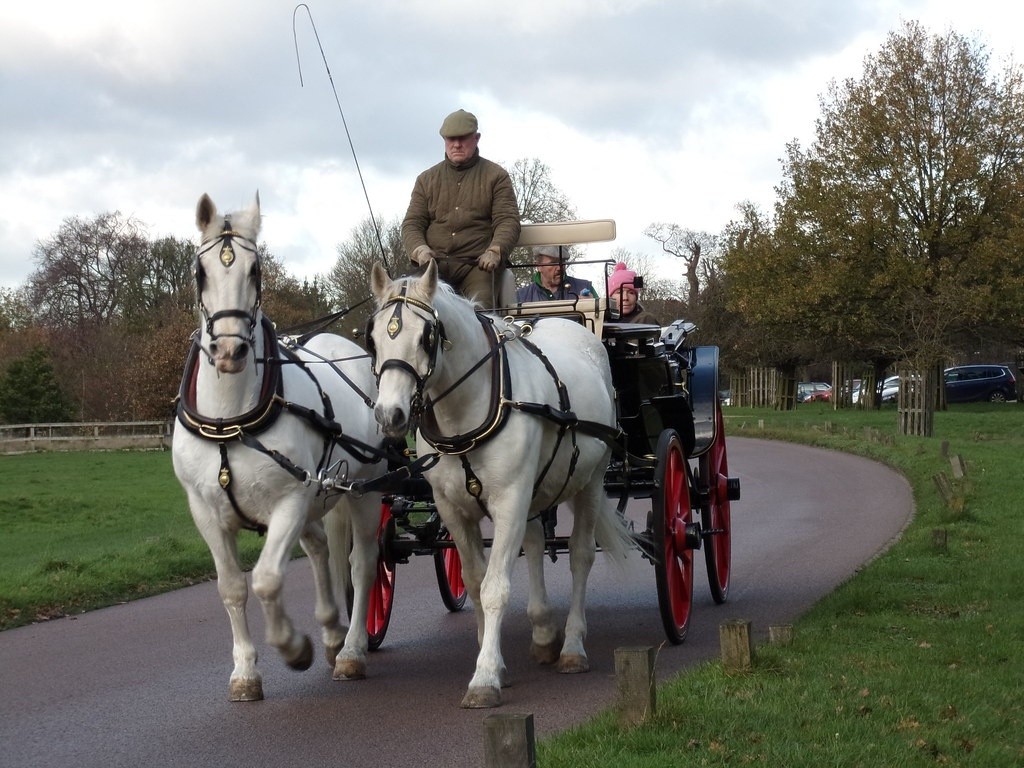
[608,262,639,297]
[440,109,478,137]
[532,246,570,259]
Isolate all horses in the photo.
[170,189,392,701]
[371,259,659,709]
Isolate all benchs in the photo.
[505,219,615,341]
[689,346,719,458]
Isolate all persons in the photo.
[401,109,520,312]
[606,262,661,346]
[515,244,600,303]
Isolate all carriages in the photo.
[168,189,741,711]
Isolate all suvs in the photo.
[942,364,1018,404]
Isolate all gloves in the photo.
[476,246,502,272]
[411,245,436,267]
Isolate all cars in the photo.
[841,374,923,405]
[716,388,776,408]
[796,381,832,404]
